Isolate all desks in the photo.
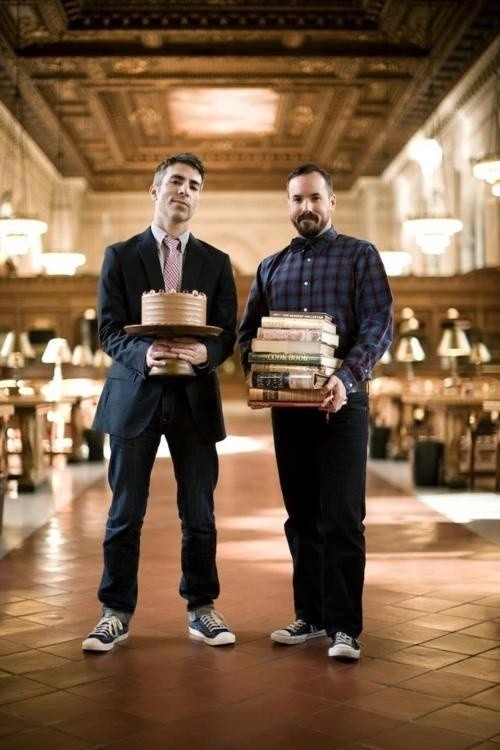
[368,391,500,491]
[0,389,103,494]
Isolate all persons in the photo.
[82,152,235,652]
[236,162,394,661]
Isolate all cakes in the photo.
[141,289,209,326]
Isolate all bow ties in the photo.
[291,238,324,256]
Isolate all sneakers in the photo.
[328,632,366,659]
[189,609,236,646]
[270,619,327,644]
[82,615,128,652]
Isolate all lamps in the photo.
[0,0,86,276]
[379,11,500,278]
[1,331,112,396]
[377,328,492,389]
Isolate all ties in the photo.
[163,236,180,292]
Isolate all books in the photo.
[248,310,349,408]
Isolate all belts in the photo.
[347,380,369,393]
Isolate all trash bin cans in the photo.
[414,442,443,486]
[370,428,391,459]
